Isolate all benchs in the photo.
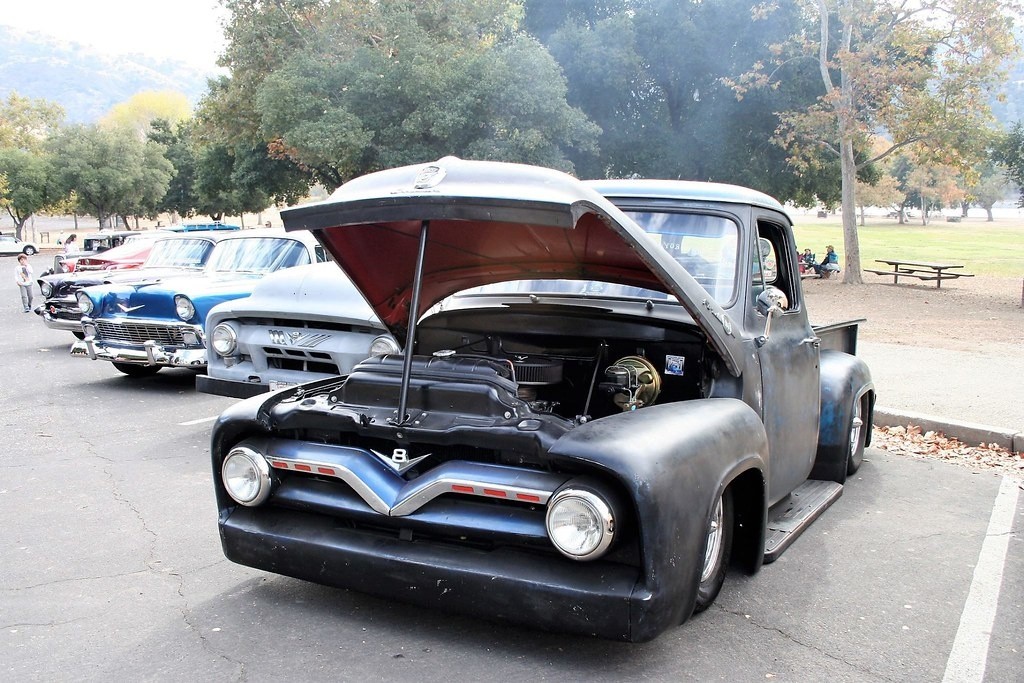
[863,268,975,287]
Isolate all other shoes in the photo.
[25,307,29,312]
[29,306,31,310]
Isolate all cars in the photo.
[0,233,40,257]
[207,153,875,644]
[193,261,401,400]
[33,221,334,351]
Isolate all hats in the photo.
[826,245,833,250]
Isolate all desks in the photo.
[875,259,963,287]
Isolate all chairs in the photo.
[802,253,816,273]
[817,254,841,279]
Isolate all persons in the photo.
[64,234,80,253]
[14,253,34,313]
[794,245,838,280]
